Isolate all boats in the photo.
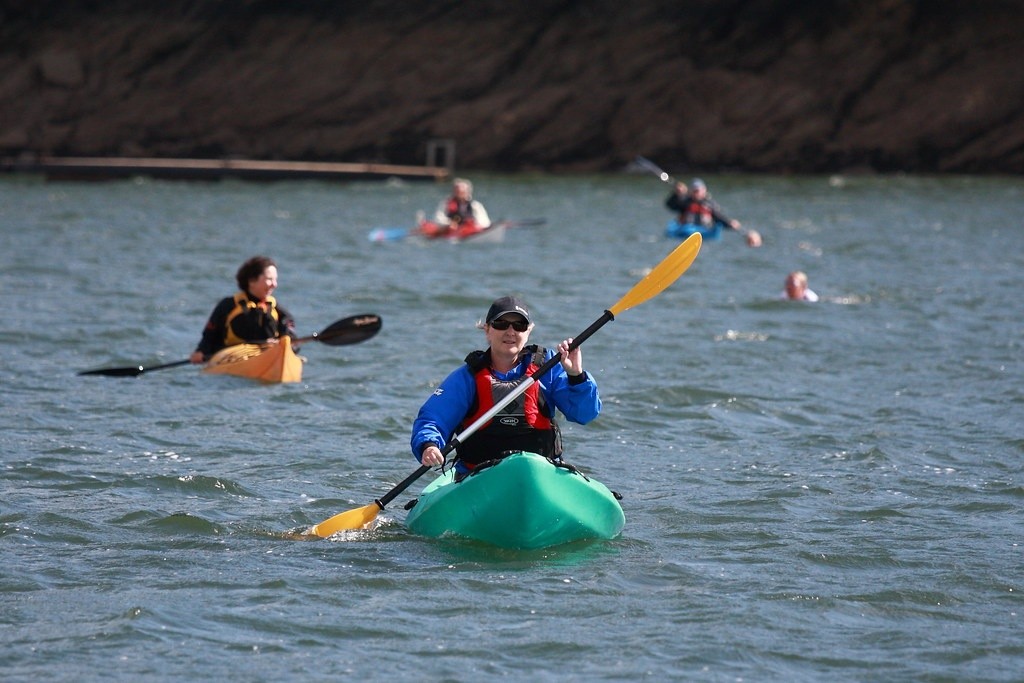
[662,218,723,242]
[403,451,628,550]
[202,335,306,382]
[411,207,511,243]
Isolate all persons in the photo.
[665,178,739,229]
[435,177,491,229]
[411,296,603,480]
[783,271,819,301]
[189,256,308,366]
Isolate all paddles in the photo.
[285,232,705,537]
[483,219,547,226]
[639,159,758,240]
[74,315,382,370]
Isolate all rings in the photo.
[424,457,429,461]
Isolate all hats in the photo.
[485,296,531,324]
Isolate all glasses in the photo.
[490,320,529,332]
